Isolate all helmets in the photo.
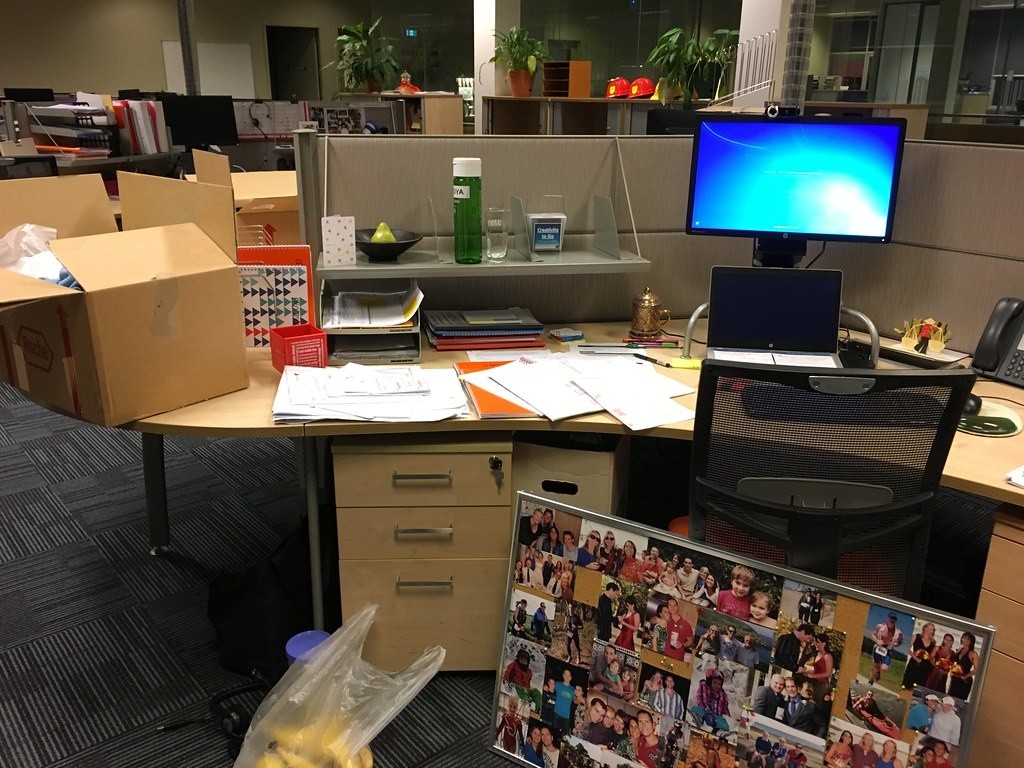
[605,77,630,98]
[673,721,682,730]
[706,668,725,687]
[516,648,530,671]
[650,77,682,100]
[627,78,656,99]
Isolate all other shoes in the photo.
[576,658,581,664]
[566,654,572,664]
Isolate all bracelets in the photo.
[682,643,684,647]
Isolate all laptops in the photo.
[706,266,843,369]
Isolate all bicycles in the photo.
[662,740,679,768]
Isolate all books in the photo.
[424,307,545,351]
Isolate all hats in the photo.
[925,694,940,703]
[942,696,956,707]
[702,733,714,746]
[888,612,897,620]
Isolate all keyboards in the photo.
[741,384,944,428]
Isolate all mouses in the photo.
[962,393,982,417]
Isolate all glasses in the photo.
[590,534,601,542]
[605,536,614,540]
[729,630,736,634]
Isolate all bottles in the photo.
[452,158,483,264]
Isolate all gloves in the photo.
[504,679,512,692]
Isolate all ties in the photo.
[548,564,550,567]
[791,700,796,717]
[527,569,530,582]
[552,581,558,594]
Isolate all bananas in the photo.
[255,724,374,768]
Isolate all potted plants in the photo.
[644,27,740,108]
[488,26,551,97]
[321,16,400,93]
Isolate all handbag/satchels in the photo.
[232,601,446,768]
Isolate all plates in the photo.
[629,330,662,341]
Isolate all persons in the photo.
[914,318,938,355]
[492,508,978,768]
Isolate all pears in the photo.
[370,221,397,243]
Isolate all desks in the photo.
[803,100,930,140]
[122,315,1024,511]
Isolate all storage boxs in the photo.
[513,431,629,518]
[0,149,248,429]
[183,171,303,245]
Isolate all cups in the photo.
[484,208,510,259]
[631,288,671,336]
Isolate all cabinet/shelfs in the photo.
[543,61,590,98]
[965,505,1023,768]
[330,432,514,671]
[327,92,465,139]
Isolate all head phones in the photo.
[249,101,270,126]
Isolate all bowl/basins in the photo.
[355,228,423,263]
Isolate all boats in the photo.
[848,688,901,740]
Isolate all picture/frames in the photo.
[487,488,996,768]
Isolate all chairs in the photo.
[668,358,978,604]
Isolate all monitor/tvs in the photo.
[686,113,908,244]
[170,95,239,153]
